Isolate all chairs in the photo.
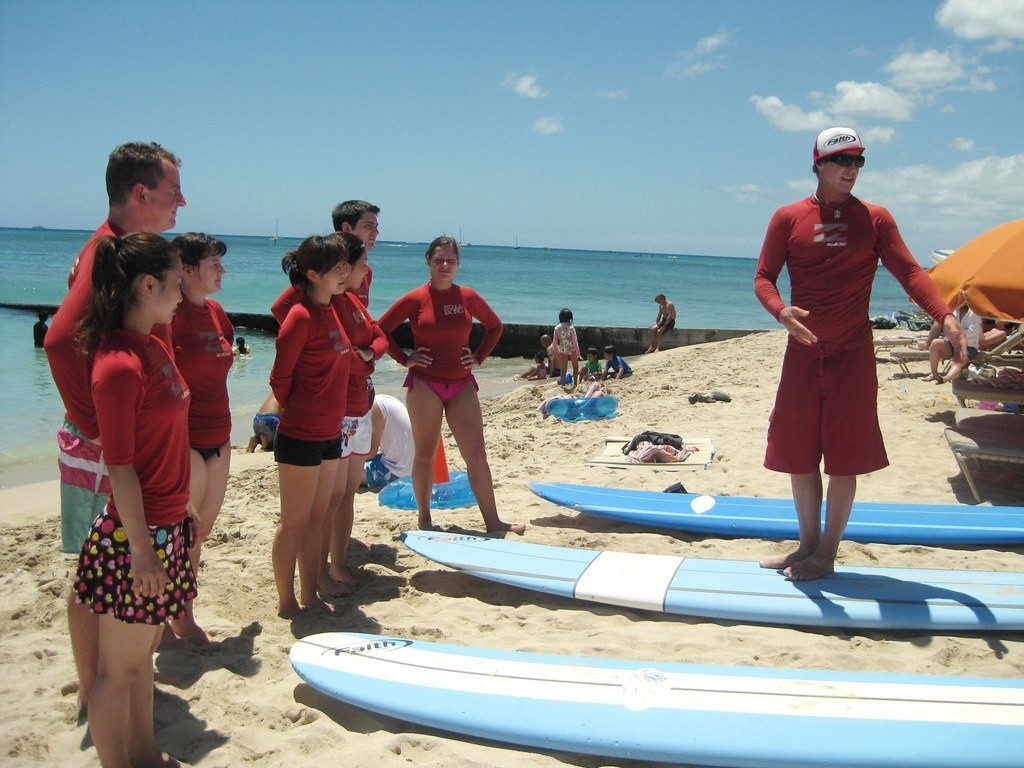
[874,330,1024,382]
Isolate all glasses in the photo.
[824,153,865,167]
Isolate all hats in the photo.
[813,127,865,164]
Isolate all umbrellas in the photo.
[907,218,1024,323]
[928,248,956,264]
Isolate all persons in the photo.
[907,301,1015,384]
[269,236,351,621]
[755,128,967,581]
[316,232,389,597]
[33,309,47,347]
[520,334,563,381]
[570,345,634,382]
[46,142,188,714]
[162,233,234,649]
[73,233,196,768]
[233,337,250,356]
[645,294,677,354]
[552,308,582,386]
[271,201,380,330]
[247,391,414,489]
[377,236,526,535]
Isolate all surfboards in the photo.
[401,530,1024,631]
[527,478,1024,544]
[289,631,1024,768]
